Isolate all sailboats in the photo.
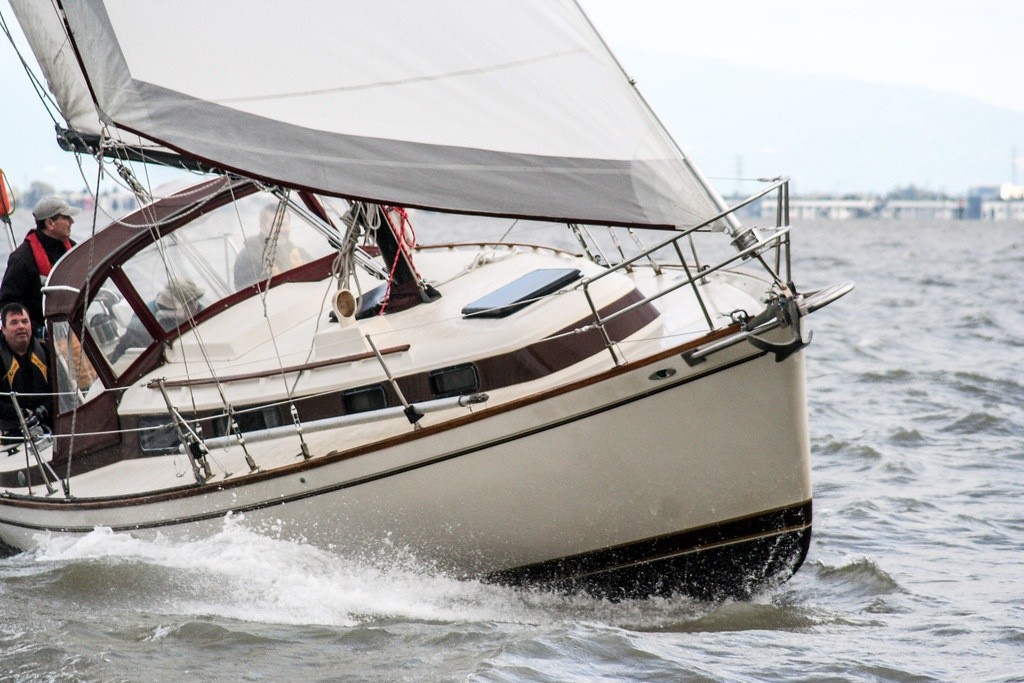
[0,0,856,605]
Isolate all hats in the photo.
[33,194,81,221]
[157,278,205,310]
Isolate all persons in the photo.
[1,192,83,347]
[0,300,57,451]
[113,277,212,362]
[233,202,315,299]
